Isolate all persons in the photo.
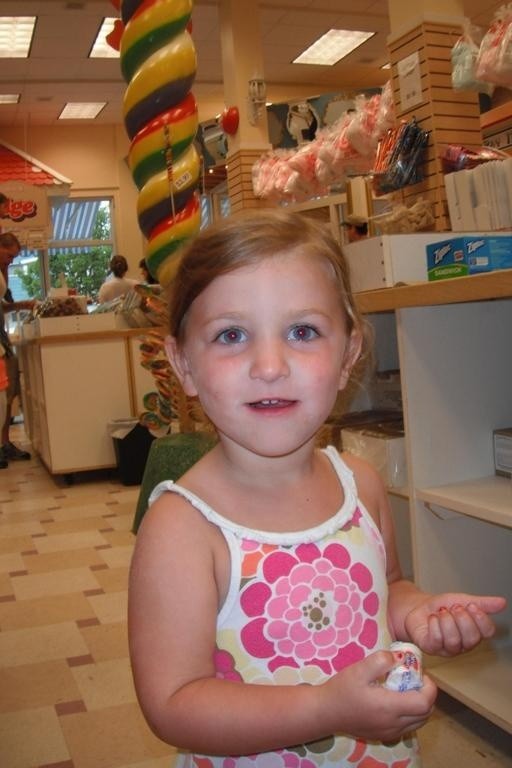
[139,257,159,283]
[0,231,36,459]
[0,269,11,466]
[125,209,507,767]
[96,255,140,303]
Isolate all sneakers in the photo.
[0,441,31,470]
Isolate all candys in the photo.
[132,284,209,431]
[120,1,202,289]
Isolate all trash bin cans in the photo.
[108,416,157,486]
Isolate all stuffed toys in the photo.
[470,17,512,92]
[449,38,497,94]
[248,81,397,204]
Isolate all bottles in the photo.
[384,639,425,692]
